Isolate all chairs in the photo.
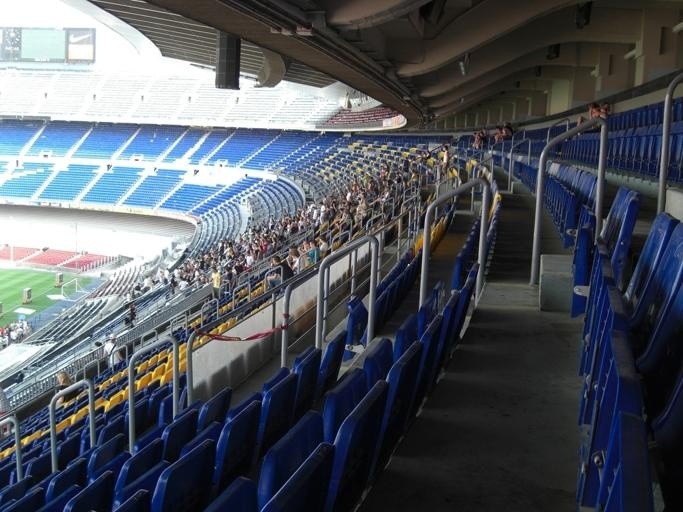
[0,66,680,512]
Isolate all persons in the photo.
[473,121,514,149]
[576,102,611,127]
[124,145,450,326]
[56,372,78,405]
[104,333,123,367]
[1,319,33,347]
